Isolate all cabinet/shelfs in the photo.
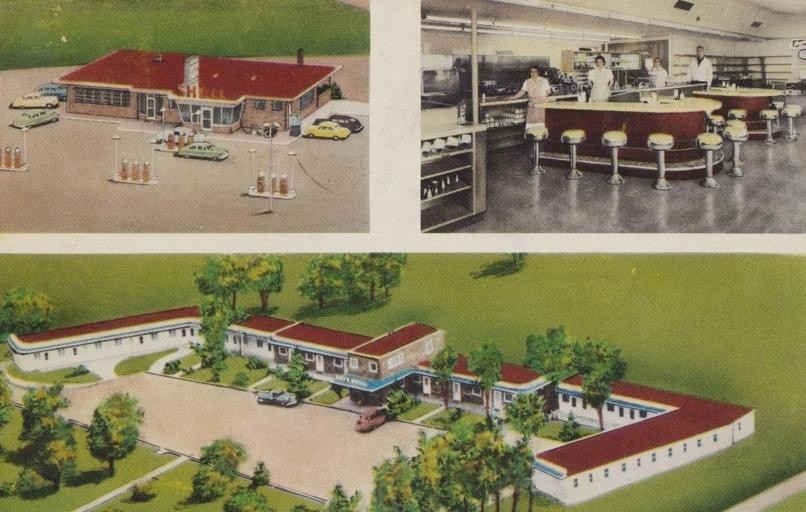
[673,54,792,85]
[420,129,487,232]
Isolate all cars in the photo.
[258,389,297,408]
[350,388,384,406]
[354,407,388,432]
[10,83,68,109]
[156,125,230,162]
[10,109,60,129]
[302,113,364,141]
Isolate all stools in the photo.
[522,124,725,192]
[708,100,803,178]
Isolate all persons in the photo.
[507,64,552,141]
[584,50,616,105]
[650,56,667,87]
[687,45,713,87]
